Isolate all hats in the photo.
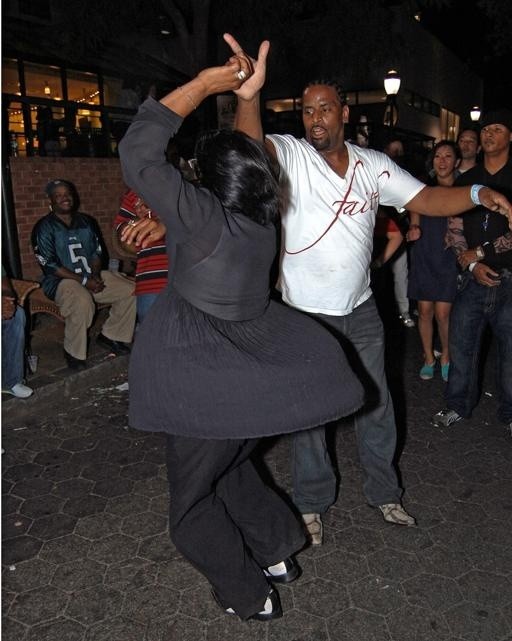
[46,180,71,197]
[479,109,512,134]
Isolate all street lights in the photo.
[467,106,481,125]
[382,71,401,142]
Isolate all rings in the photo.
[128,218,136,228]
[237,70,247,82]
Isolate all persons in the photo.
[221,29,511,549]
[372,207,404,273]
[427,107,512,432]
[118,52,363,622]
[112,190,170,323]
[35,105,66,157]
[404,140,463,384]
[30,178,133,370]
[65,117,108,158]
[379,135,415,327]
[428,127,483,181]
[1,263,34,398]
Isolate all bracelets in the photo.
[80,274,88,288]
[466,262,479,273]
[469,183,487,206]
[178,84,197,112]
[475,243,486,263]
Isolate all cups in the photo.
[29,355,38,372]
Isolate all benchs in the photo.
[27,276,138,375]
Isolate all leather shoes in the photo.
[366,502,416,528]
[263,555,298,583]
[299,513,322,548]
[209,585,283,620]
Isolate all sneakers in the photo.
[95,333,131,356]
[398,314,416,328]
[2,384,33,399]
[418,359,437,380]
[441,363,450,383]
[430,408,466,431]
[501,423,512,446]
[62,348,87,370]
